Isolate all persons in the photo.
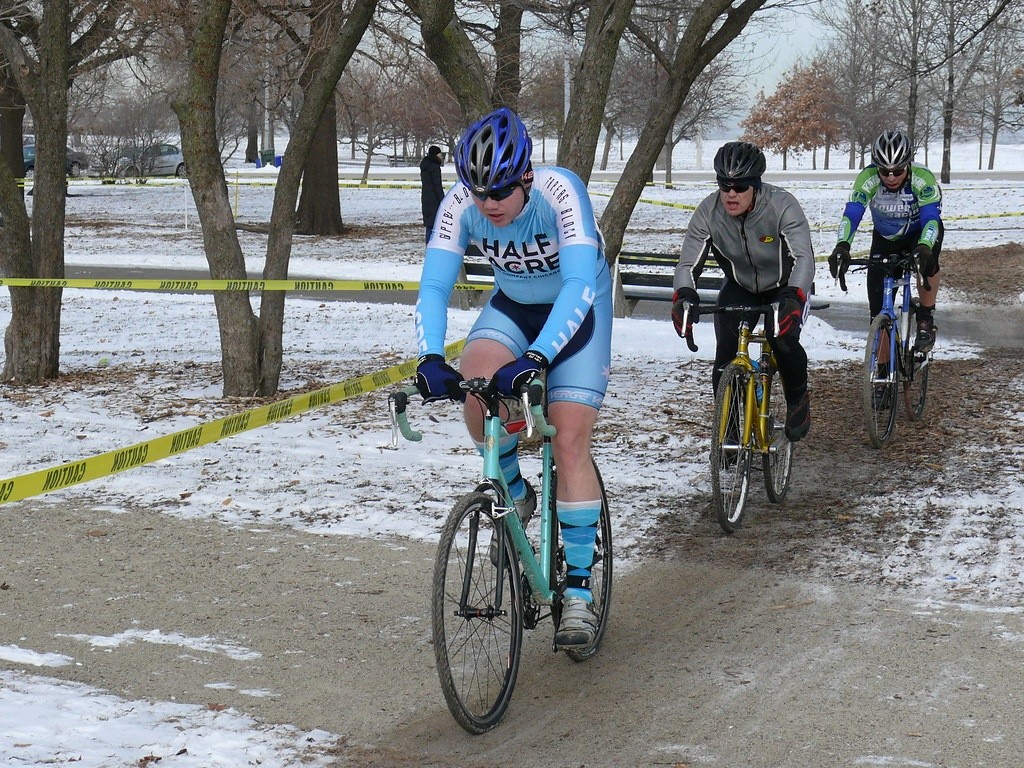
[415,109,613,650]
[670,142,815,470]
[419,146,444,244]
[828,130,945,409]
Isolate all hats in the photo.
[429,146,441,156]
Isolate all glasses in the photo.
[718,180,749,193]
[471,182,520,201]
[879,168,904,177]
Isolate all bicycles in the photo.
[834,250,932,451]
[387,368,613,733]
[676,297,793,535]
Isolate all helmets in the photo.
[871,129,913,170]
[454,107,532,193]
[714,142,766,179]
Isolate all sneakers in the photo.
[489,478,536,568]
[555,596,597,647]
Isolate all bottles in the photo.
[746,371,763,408]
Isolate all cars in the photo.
[107,143,187,179]
[22,144,89,181]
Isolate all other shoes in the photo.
[709,443,740,465]
[872,379,887,410]
[915,316,935,352]
[785,380,809,442]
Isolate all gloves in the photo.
[491,349,548,394]
[777,286,807,338]
[910,244,932,274]
[672,287,700,338]
[417,355,466,402]
[827,241,850,279]
